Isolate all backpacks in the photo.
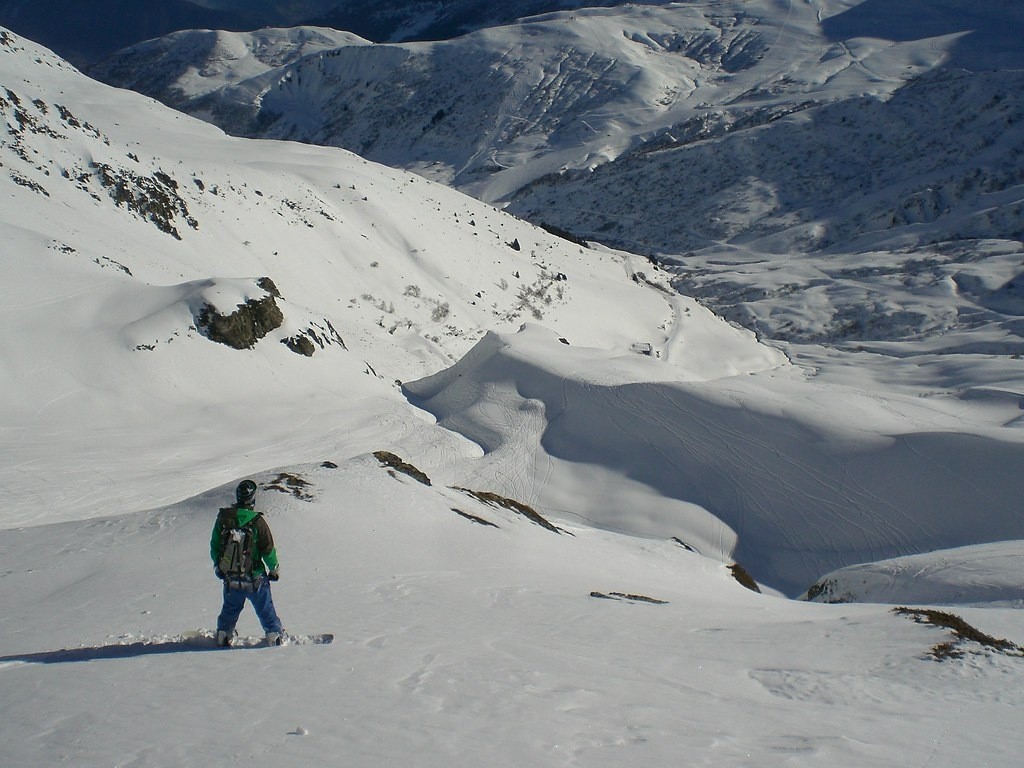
[218,508,263,580]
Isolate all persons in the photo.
[212,481,288,648]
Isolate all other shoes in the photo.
[270,631,289,646]
[218,630,235,647]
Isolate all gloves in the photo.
[214,564,223,579]
[268,570,279,581]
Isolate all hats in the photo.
[236,480,257,501]
